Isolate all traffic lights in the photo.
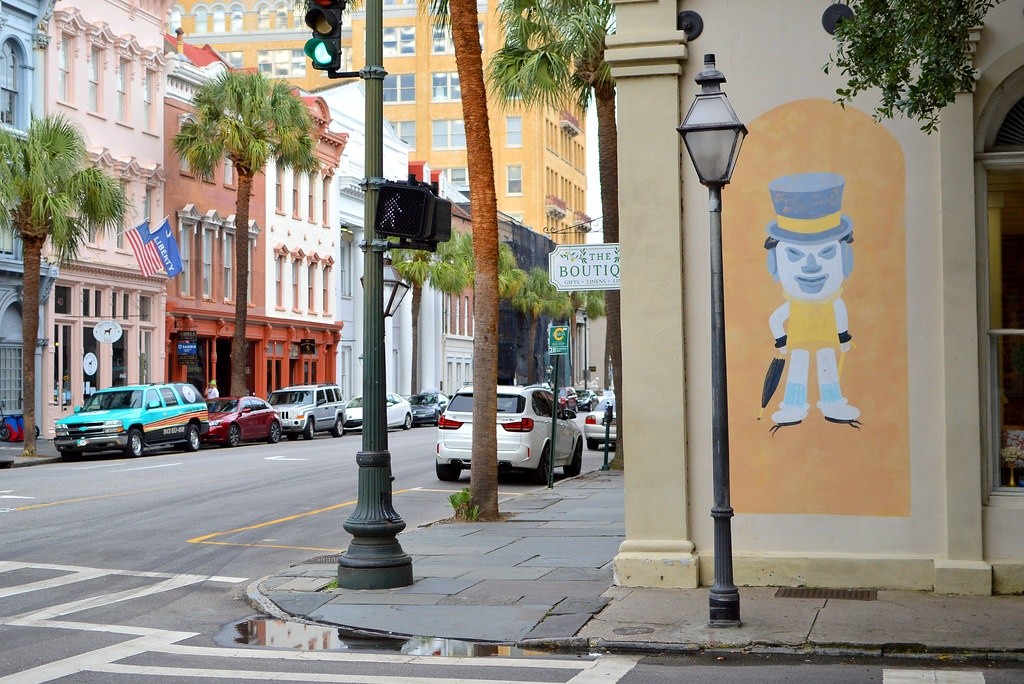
[373,174,451,253]
[302,0,346,71]
[582,310,588,390]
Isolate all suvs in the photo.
[261,383,346,440]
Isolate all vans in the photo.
[53,383,209,458]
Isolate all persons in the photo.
[205,380,219,399]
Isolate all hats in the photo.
[208,379,216,384]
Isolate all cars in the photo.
[186,395,282,447]
[336,393,413,431]
[406,371,599,427]
[436,383,582,483]
[585,397,616,450]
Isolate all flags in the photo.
[150,217,183,277]
[124,220,163,277]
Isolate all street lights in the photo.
[675,54,751,629]
